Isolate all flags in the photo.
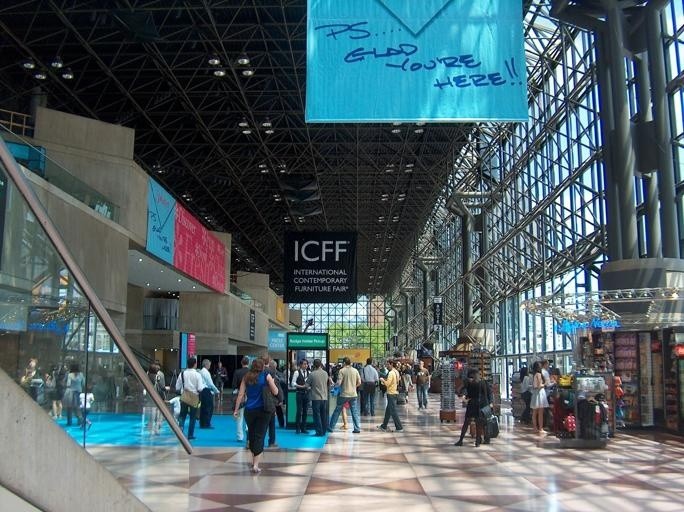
[144,176,177,267]
[281,228,358,304]
[305,0,530,126]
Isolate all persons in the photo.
[140,364,169,434]
[375,357,406,433]
[230,357,250,443]
[302,359,332,435]
[215,358,228,403]
[245,354,284,450]
[21,356,95,431]
[232,356,280,472]
[164,389,180,425]
[452,367,494,446]
[327,356,362,433]
[173,356,208,441]
[195,358,220,429]
[518,357,556,434]
[122,370,132,400]
[290,357,311,435]
[262,357,431,427]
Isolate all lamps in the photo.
[238,116,255,138]
[21,56,49,83]
[51,57,77,83]
[256,160,310,227]
[154,165,283,301]
[233,54,255,79]
[207,54,227,80]
[519,284,683,327]
[362,120,428,301]
[258,118,276,139]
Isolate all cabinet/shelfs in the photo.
[660,325,678,433]
[469,351,494,386]
[546,384,610,450]
[596,330,644,433]
[577,333,597,373]
[673,351,684,440]
[509,369,528,418]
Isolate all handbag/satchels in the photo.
[364,382,375,393]
[263,387,276,413]
[397,393,408,404]
[180,390,199,408]
[480,405,499,437]
